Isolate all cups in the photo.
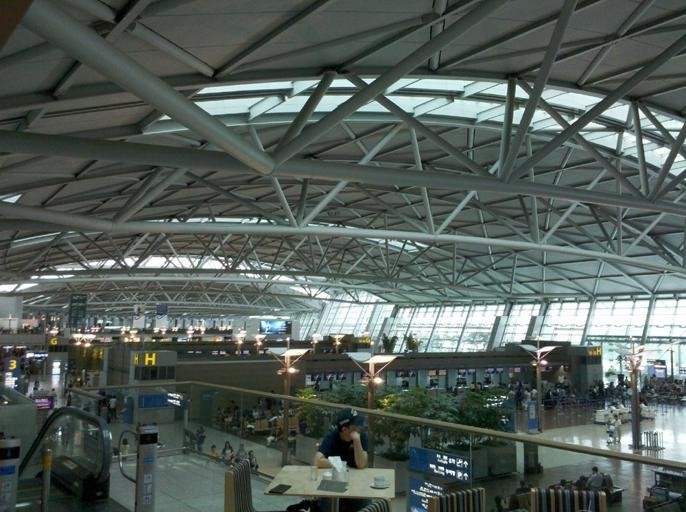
[375,475,385,486]
[322,471,333,480]
[311,466,318,480]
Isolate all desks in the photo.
[264,462,397,512]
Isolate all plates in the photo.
[370,483,392,489]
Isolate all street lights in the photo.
[342,340,405,468]
[249,333,267,357]
[608,334,652,451]
[232,332,247,357]
[516,335,564,434]
[263,336,315,467]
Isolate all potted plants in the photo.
[293,385,516,495]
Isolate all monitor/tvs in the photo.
[487,368,495,374]
[496,367,504,373]
[458,368,466,376]
[407,368,417,377]
[437,369,447,376]
[513,366,521,374]
[310,372,346,382]
[427,369,437,377]
[467,368,476,375]
[395,369,406,377]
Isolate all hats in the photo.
[337,408,366,427]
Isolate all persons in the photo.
[515,480,531,494]
[470,373,686,442]
[196,389,294,470]
[585,466,603,490]
[24,360,118,420]
[313,407,370,469]
[528,482,536,488]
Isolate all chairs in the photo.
[223,459,260,512]
[426,486,485,512]
[525,486,608,512]
[360,498,394,512]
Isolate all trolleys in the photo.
[606,423,618,445]
